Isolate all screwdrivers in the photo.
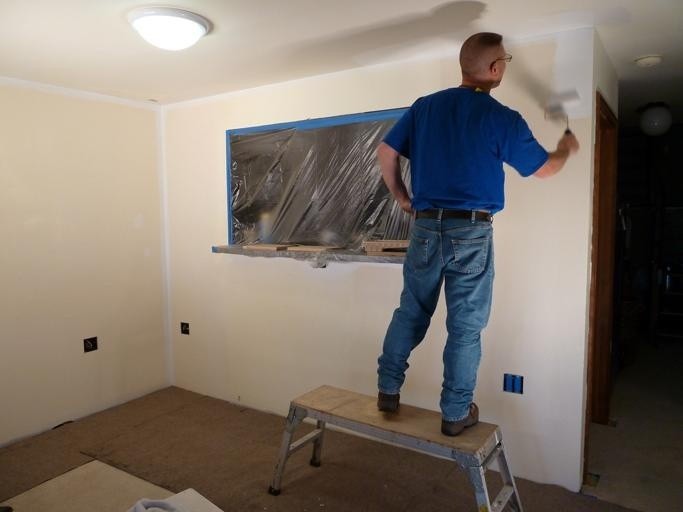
[566,115,571,134]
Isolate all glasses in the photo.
[490,53,512,69]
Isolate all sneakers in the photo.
[378,393,400,412]
[441,402,479,436]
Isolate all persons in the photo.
[373,32,581,435]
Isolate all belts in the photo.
[416,209,491,222]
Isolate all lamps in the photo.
[126,7,211,53]
[634,101,673,137]
